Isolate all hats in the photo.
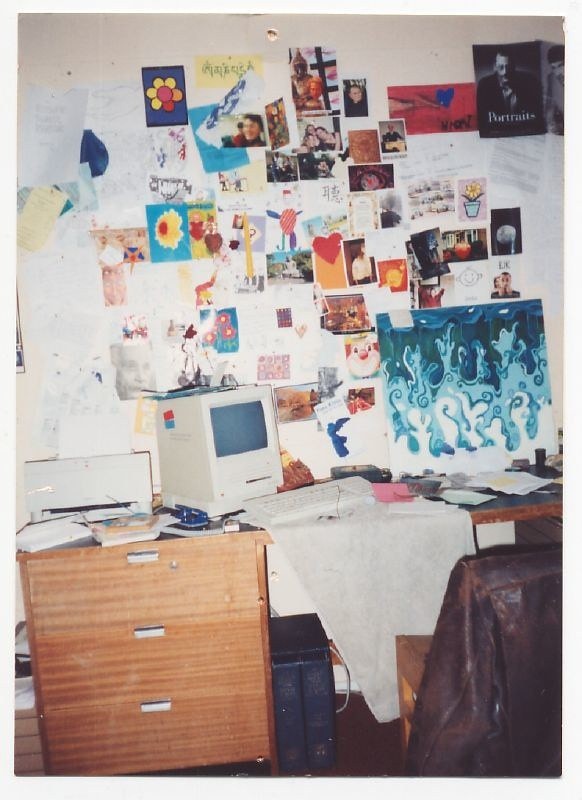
[292,48,306,65]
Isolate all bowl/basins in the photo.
[400,478,441,496]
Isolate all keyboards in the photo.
[241,475,374,526]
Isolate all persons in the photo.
[384,123,405,142]
[424,288,445,310]
[301,123,343,151]
[266,152,294,183]
[543,44,564,133]
[344,84,368,117]
[426,234,442,265]
[309,389,320,412]
[290,50,313,111]
[351,244,372,285]
[476,48,544,138]
[303,77,324,110]
[232,114,265,147]
[346,394,372,414]
[490,272,520,299]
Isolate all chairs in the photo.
[395,634,438,752]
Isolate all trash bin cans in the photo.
[268,611,338,777]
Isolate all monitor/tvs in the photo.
[156,384,285,507]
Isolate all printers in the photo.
[24,451,153,526]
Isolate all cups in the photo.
[535,448,546,467]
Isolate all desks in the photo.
[428,464,562,524]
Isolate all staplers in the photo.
[175,504,208,527]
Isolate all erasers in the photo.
[224,520,240,532]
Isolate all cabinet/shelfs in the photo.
[16,528,265,776]
